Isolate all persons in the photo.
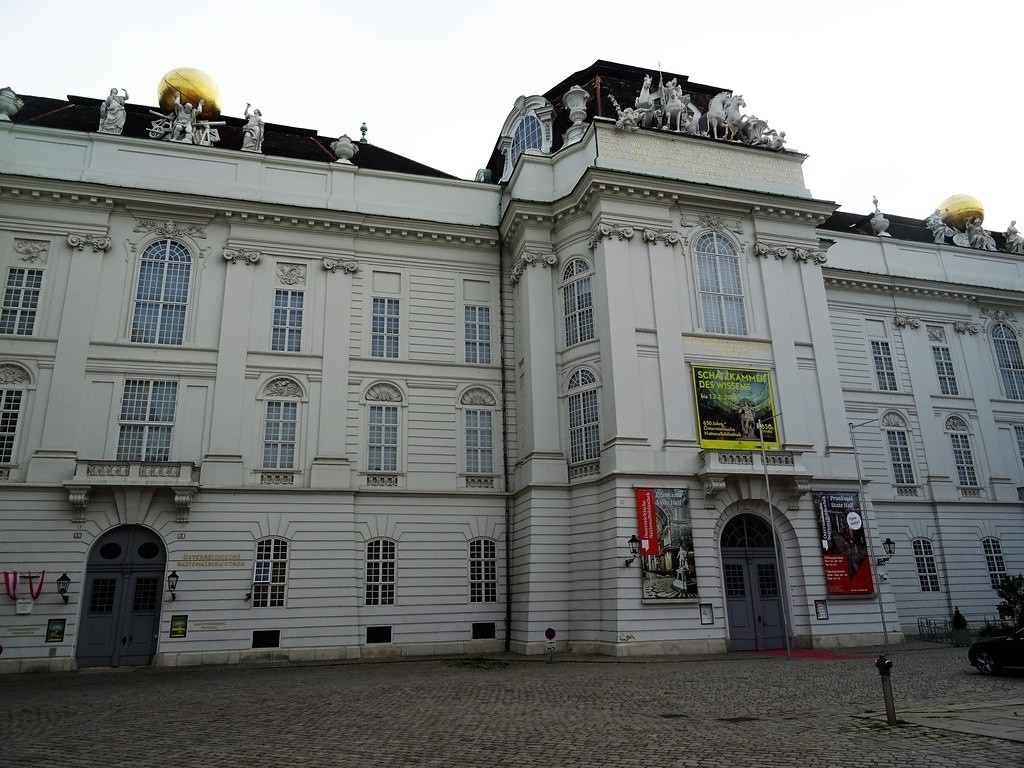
[675,546,687,567]
[966,217,991,250]
[926,208,949,243]
[242,102,265,153]
[105,87,129,128]
[1006,220,1021,252]
[739,401,756,434]
[750,119,786,150]
[172,92,204,143]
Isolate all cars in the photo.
[967,626,1024,679]
